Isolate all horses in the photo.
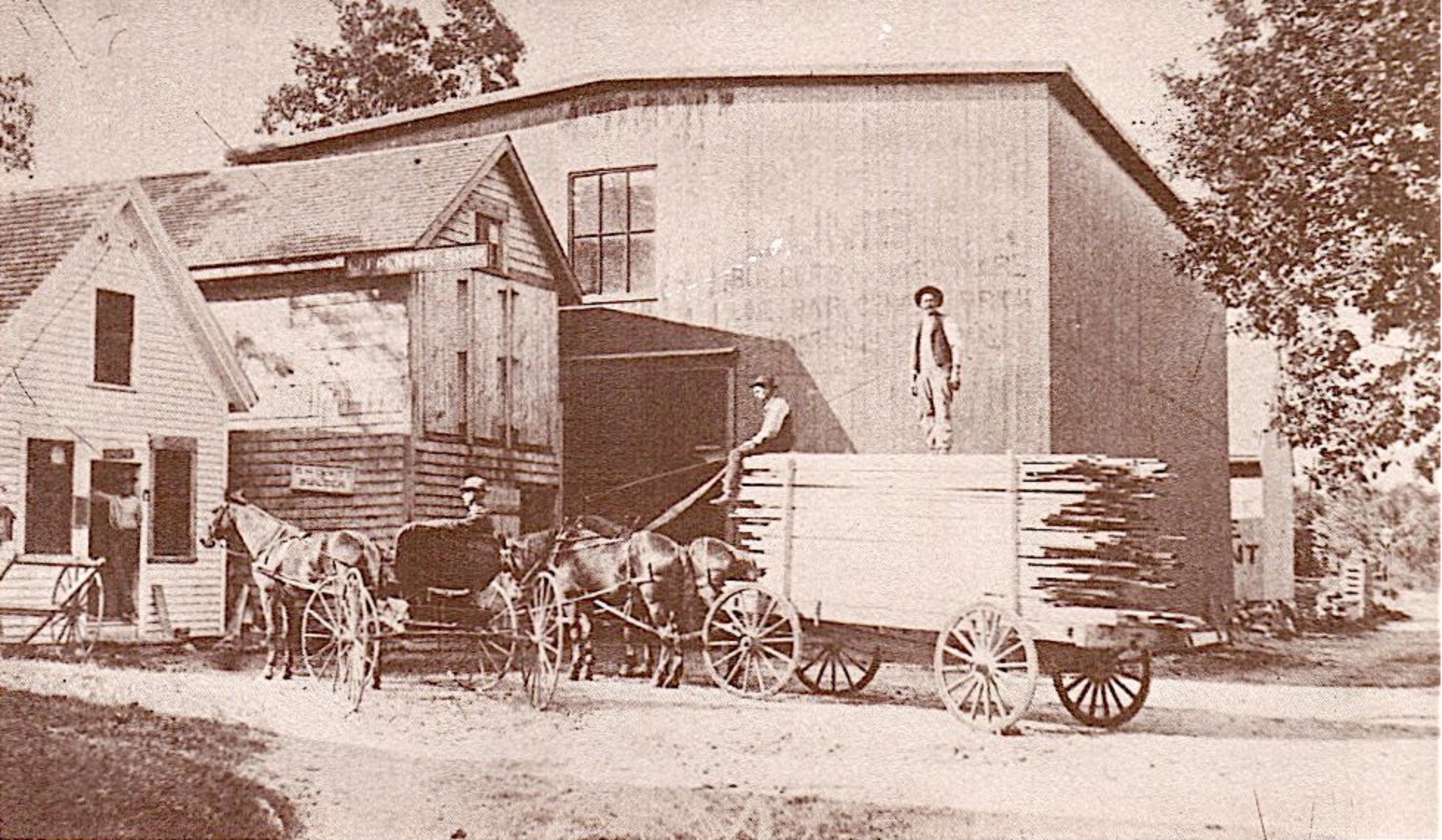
[498,510,757,689]
[199,486,384,690]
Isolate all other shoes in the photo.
[708,493,735,505]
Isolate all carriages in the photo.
[197,490,521,700]
[490,506,1233,728]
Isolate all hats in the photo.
[916,286,943,308]
[747,374,778,389]
[461,475,492,493]
[117,469,137,483]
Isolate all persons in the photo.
[459,476,488,518]
[90,472,142,622]
[910,286,961,453]
[709,375,796,506]
